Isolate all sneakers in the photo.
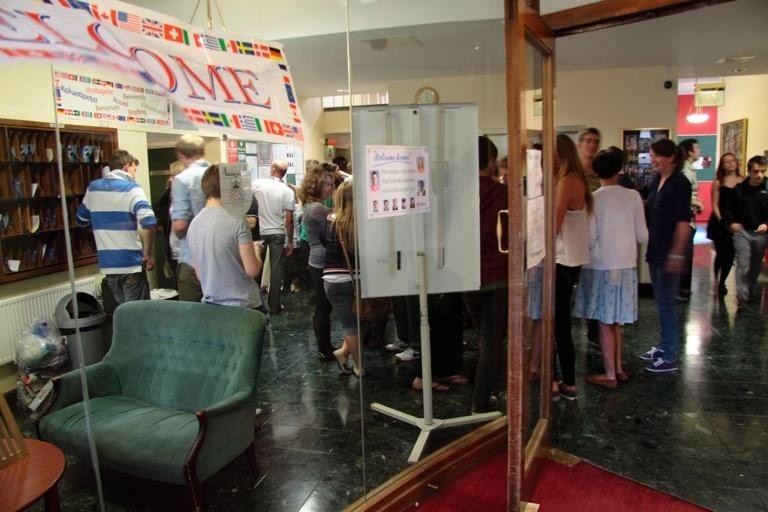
[718,285,727,294]
[677,289,690,300]
[638,345,665,360]
[645,357,679,372]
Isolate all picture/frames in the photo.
[717,117,749,175]
[619,124,672,194]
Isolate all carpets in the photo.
[401,446,716,511]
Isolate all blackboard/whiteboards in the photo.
[621,128,672,203]
[719,117,748,180]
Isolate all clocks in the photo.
[414,86,440,104]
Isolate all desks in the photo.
[0,437,66,511]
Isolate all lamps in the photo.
[687,78,710,123]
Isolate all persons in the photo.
[707,153,744,295]
[678,139,703,303]
[638,139,691,373]
[160,125,614,402]
[725,156,768,313]
[73,150,156,305]
[577,147,648,389]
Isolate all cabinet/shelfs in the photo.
[0,117,120,285]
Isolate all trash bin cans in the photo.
[54,291,107,371]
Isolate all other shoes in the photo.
[319,339,628,401]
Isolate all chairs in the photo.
[30,297,267,512]
